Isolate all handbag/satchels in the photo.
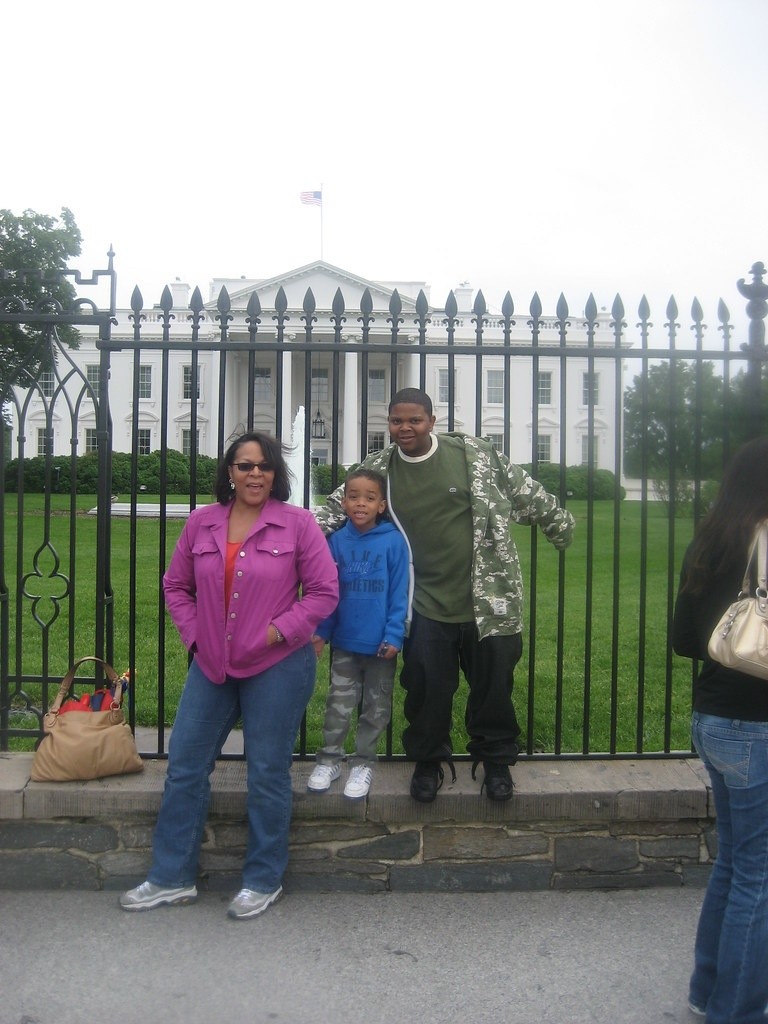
[31,657,144,782]
[708,517,768,681]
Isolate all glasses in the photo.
[227,461,276,473]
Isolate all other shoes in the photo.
[687,1002,705,1015]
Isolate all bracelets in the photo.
[273,625,284,644]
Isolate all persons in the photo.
[670,435,768,1024]
[309,468,410,799]
[119,423,340,921]
[314,388,576,805]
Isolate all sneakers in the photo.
[306,762,341,793]
[120,879,199,912]
[343,764,374,801]
[481,759,516,800]
[410,760,444,803]
[227,884,284,920]
